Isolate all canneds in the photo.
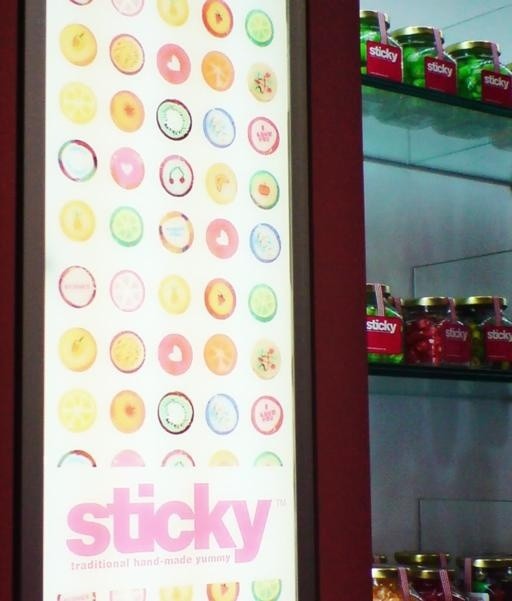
[391,25,457,95]
[443,40,511,111]
[387,295,404,315]
[359,9,403,85]
[401,296,471,370]
[366,283,404,365]
[372,551,511,601]
[455,295,511,371]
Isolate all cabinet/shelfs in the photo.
[362,73,512,385]
